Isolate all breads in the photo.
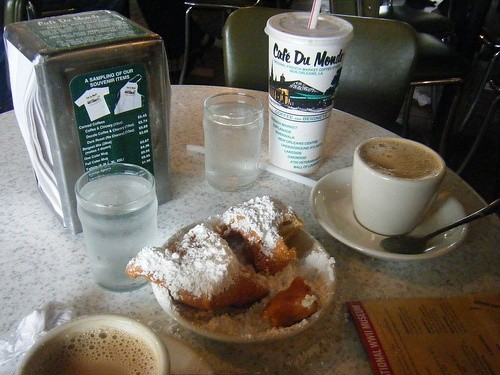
[124,196,318,329]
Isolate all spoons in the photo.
[380,199,500,254]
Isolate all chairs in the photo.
[5,0,500,175]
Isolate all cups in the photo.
[264,12,355,174]
[201,91,265,192]
[74,162,159,293]
[14,314,171,375]
[352,137,446,237]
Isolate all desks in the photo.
[0,84,500,375]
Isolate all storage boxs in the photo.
[3,8,173,238]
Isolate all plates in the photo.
[150,215,337,344]
[309,166,469,262]
[158,333,216,375]
